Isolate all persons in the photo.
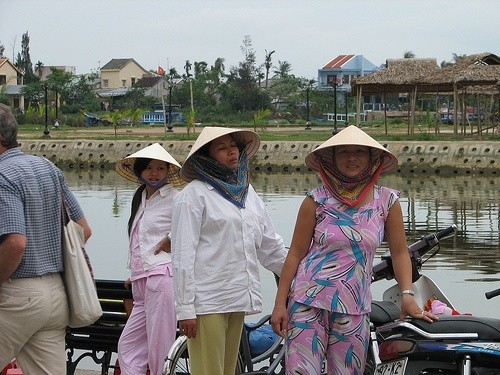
[0,102,93,375]
[268,124,441,375]
[114,143,187,375]
[172,124,290,375]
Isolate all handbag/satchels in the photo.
[61,204,103,328]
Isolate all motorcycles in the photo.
[368,225,500,375]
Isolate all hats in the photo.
[306,125,398,174]
[116,143,186,186]
[180,127,261,184]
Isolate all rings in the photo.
[422,312,424,315]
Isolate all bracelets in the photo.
[400,289,415,296]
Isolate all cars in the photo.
[435,106,486,124]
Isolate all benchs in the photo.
[63,280,272,375]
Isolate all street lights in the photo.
[168,83,174,132]
[42,86,50,138]
[305,86,313,129]
[54,89,61,128]
[332,82,343,135]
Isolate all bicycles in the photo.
[165,271,285,375]
